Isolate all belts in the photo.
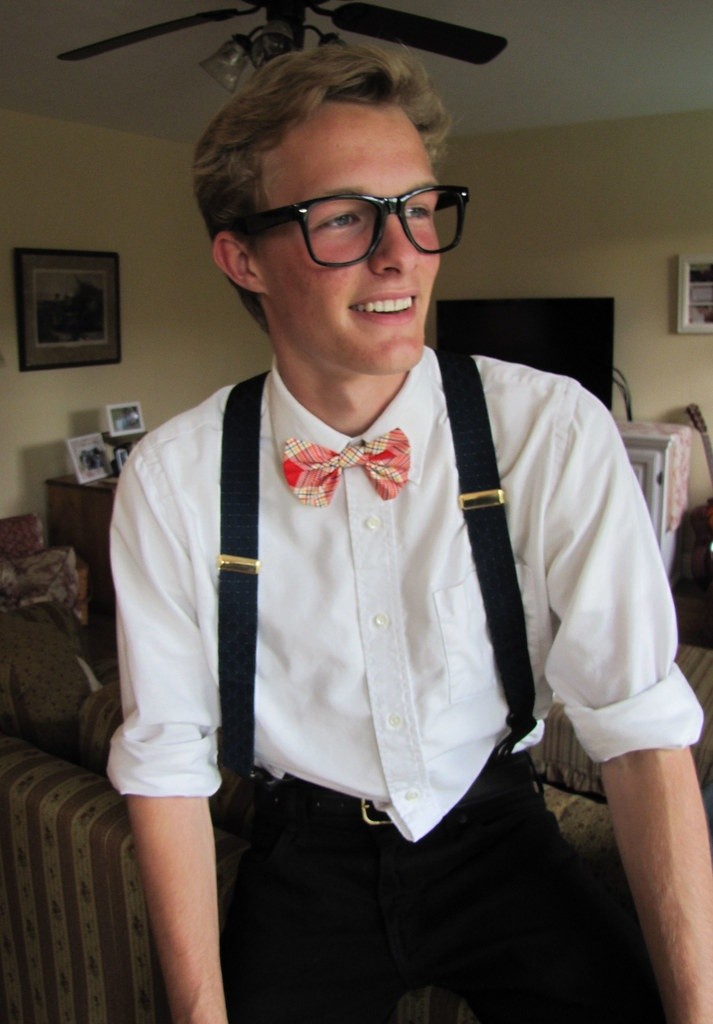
[253,754,535,827]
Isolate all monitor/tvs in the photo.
[434,297,615,414]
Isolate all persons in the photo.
[107,44,713,1024]
[80,448,104,472]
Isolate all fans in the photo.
[55,0,508,61]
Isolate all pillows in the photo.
[0,545,79,615]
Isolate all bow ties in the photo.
[283,426,412,509]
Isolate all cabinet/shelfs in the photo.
[45,472,120,663]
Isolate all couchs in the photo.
[0,512,713,1024]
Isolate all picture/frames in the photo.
[65,433,115,485]
[105,402,145,437]
[676,252,713,335]
[11,245,121,372]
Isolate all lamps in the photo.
[196,21,350,94]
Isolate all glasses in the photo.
[215,185,473,269]
